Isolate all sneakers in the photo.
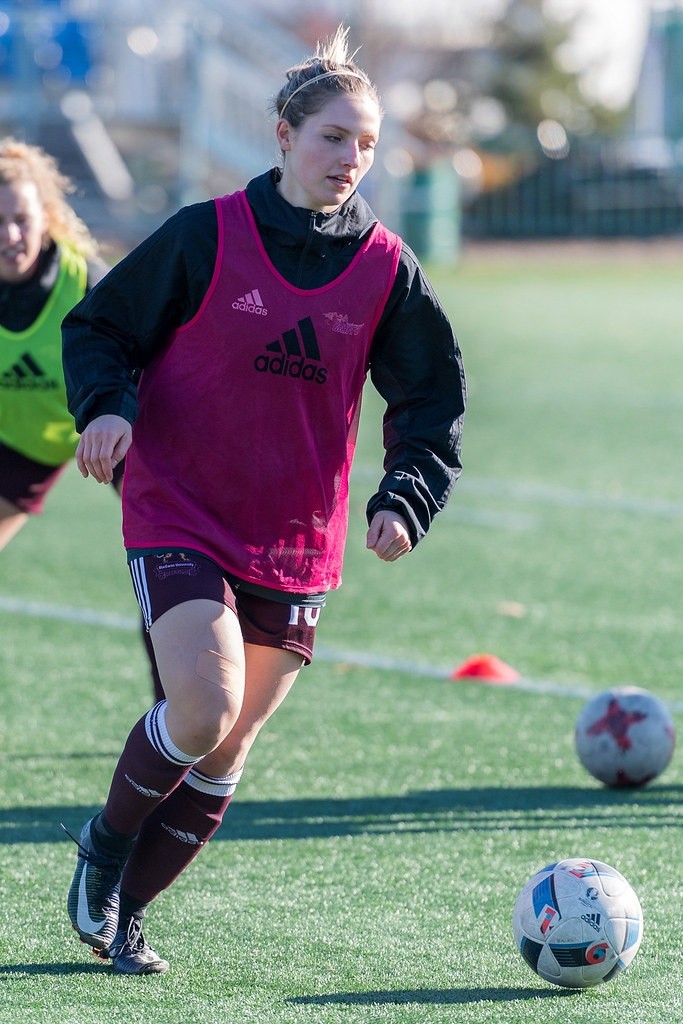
[99,914,170,975]
[61,810,130,954]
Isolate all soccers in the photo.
[574,685,677,789]
[512,858,644,987]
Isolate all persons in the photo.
[59,26,470,981]
[1,132,115,550]
